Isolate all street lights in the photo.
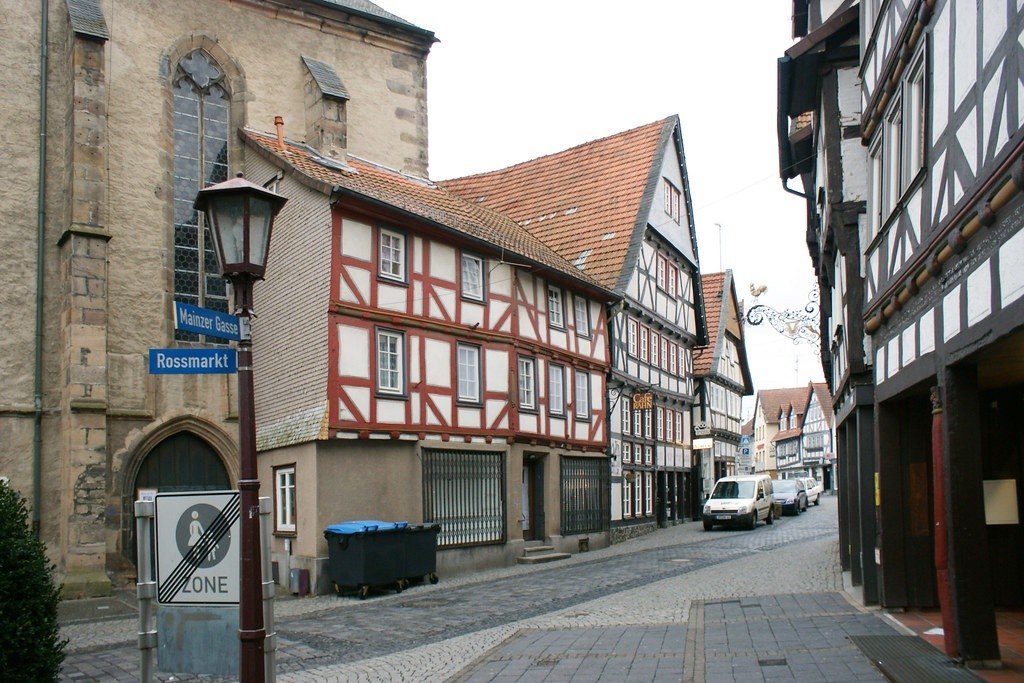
[192,171,290,682]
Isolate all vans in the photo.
[703,474,776,530]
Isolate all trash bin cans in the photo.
[405,523,441,590]
[324,519,409,599]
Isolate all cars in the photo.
[794,476,824,506]
[771,479,808,516]
